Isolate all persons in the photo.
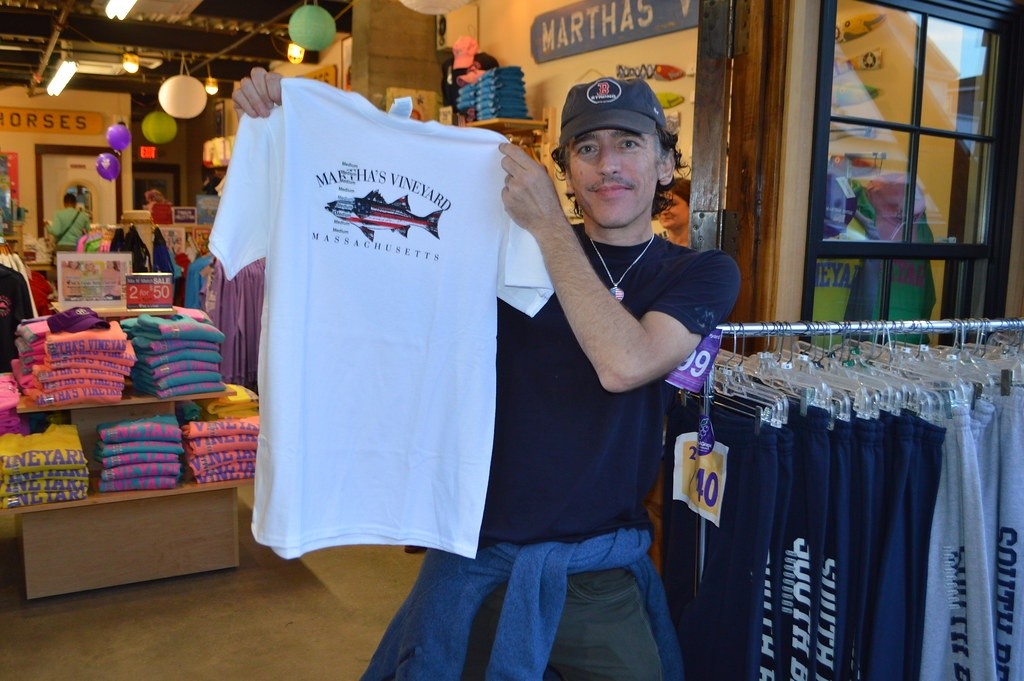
[145,189,173,224]
[656,178,691,247]
[44,193,90,251]
[231,67,742,681]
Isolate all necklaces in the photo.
[590,233,655,301]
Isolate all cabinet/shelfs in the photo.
[11,301,249,601]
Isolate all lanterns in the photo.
[158,74,207,119]
[141,112,177,144]
[289,5,336,52]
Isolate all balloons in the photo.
[96,153,120,181]
[107,124,130,150]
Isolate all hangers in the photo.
[677,317,1024,436]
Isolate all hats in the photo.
[560,77,668,145]
[453,36,499,85]
[46,308,112,334]
[867,172,925,243]
